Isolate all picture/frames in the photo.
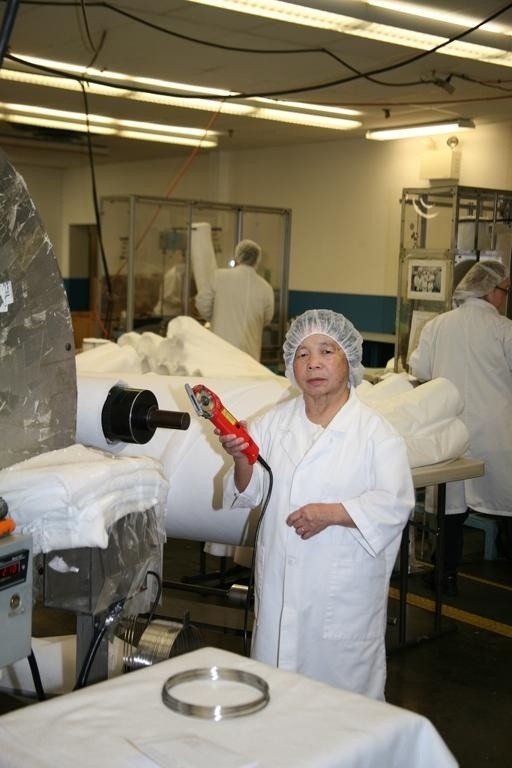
[365,119,475,141]
[402,254,452,302]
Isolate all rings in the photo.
[301,527,306,533]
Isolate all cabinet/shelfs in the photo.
[395,186,512,380]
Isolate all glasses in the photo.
[494,284,512,296]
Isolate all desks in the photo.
[386,457,486,659]
[0,647,462,768]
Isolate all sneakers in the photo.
[437,573,458,594]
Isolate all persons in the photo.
[195,239,275,365]
[407,262,512,597]
[215,307,416,700]
[155,244,194,317]
